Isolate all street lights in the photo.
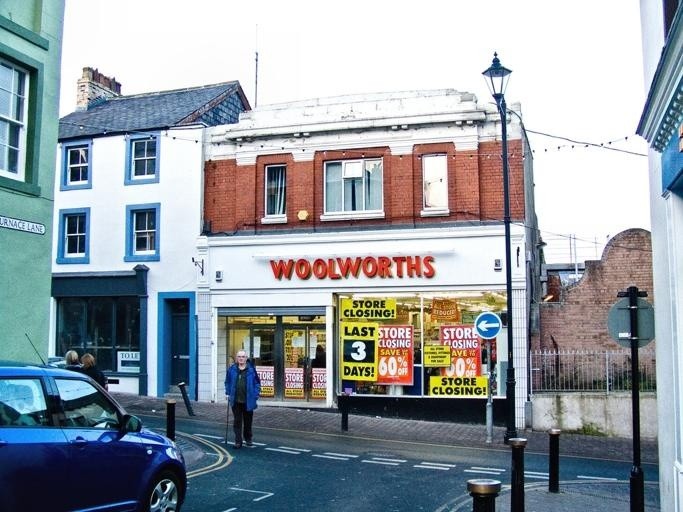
[481,51,518,444]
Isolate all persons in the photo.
[54,350,82,373]
[223,348,261,449]
[75,352,105,390]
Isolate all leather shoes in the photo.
[233,440,242,449]
[246,440,252,446]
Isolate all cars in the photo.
[1,331,187,512]
